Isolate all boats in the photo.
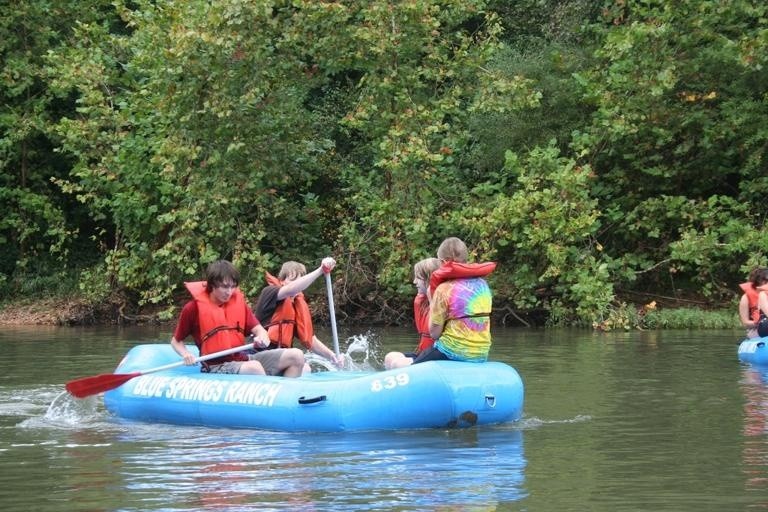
[104,343,524,432]
[738,336,768,365]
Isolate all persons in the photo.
[738,269,768,339]
[169,259,305,378]
[411,235,493,364]
[382,256,441,371]
[246,256,346,377]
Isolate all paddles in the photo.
[65,342,257,398]
